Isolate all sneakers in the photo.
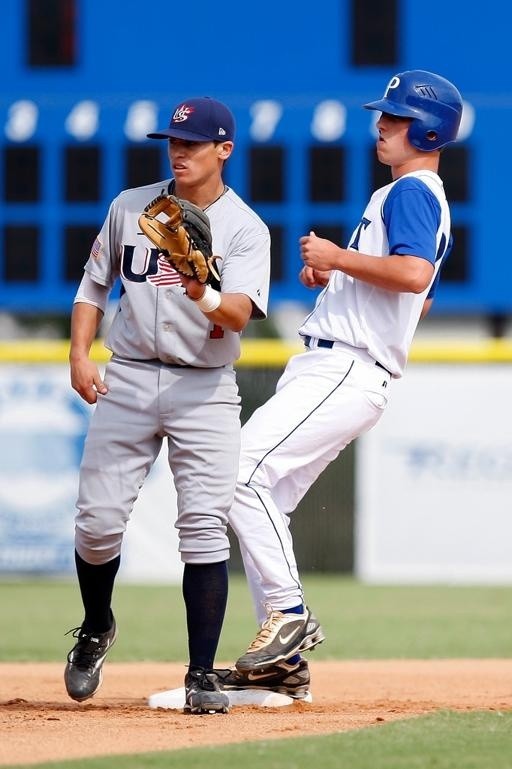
[184,605,326,715]
[64,619,117,701]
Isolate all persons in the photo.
[213,70,463,695]
[64,96,274,714]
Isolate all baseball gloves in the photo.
[137,193,223,286]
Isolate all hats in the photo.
[147,96,235,141]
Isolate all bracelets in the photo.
[190,281,222,313]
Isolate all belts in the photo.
[304,336,393,375]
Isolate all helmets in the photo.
[362,69,463,152]
